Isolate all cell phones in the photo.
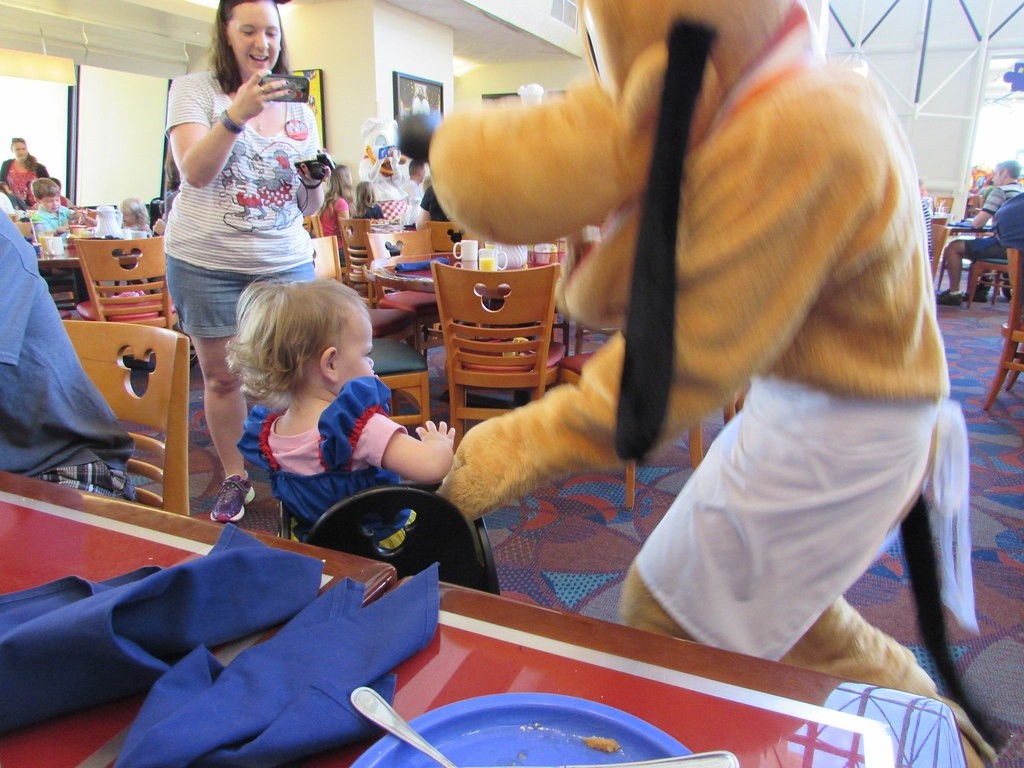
[261,74,310,103]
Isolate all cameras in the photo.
[294,152,335,180]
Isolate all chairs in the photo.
[52,196,748,509]
[931,197,1024,411]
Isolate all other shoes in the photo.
[962,291,988,302]
[936,289,963,305]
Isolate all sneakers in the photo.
[210,471,254,522]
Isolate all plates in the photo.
[346,691,694,768]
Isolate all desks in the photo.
[938,226,994,290]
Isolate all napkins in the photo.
[948,221,971,227]
[112,562,440,768]
[398,259,450,271]
[1,522,332,734]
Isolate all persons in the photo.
[164,1,331,522]
[321,164,384,268]
[0,207,137,502]
[404,158,463,244]
[917,161,1022,305]
[224,280,456,550]
[0,138,180,290]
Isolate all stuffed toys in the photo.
[428,0,1007,768]
[360,118,410,219]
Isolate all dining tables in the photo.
[366,251,589,408]
[30,240,142,310]
[1,465,970,768]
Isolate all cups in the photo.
[453,240,480,260]
[478,243,507,272]
[453,260,478,271]
[128,230,153,240]
[40,234,64,256]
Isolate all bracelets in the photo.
[221,111,245,134]
[306,180,322,189]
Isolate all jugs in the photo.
[90,203,123,238]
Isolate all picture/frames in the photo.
[392,71,444,125]
